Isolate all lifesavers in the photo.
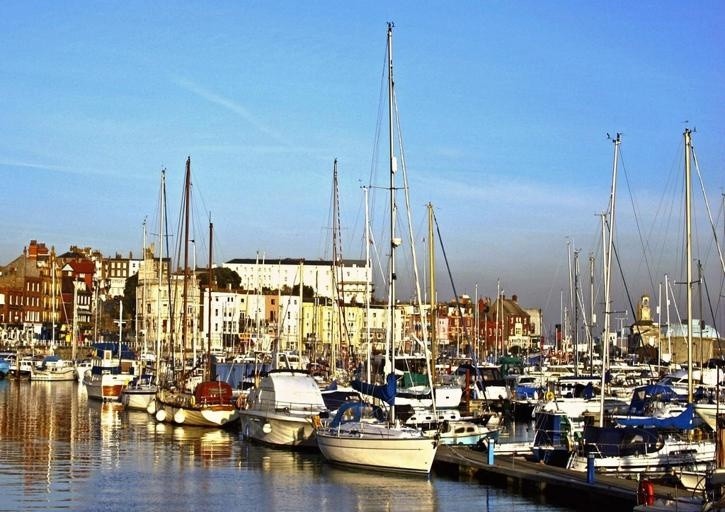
[175,395,185,406]
[190,396,195,406]
[638,480,654,506]
